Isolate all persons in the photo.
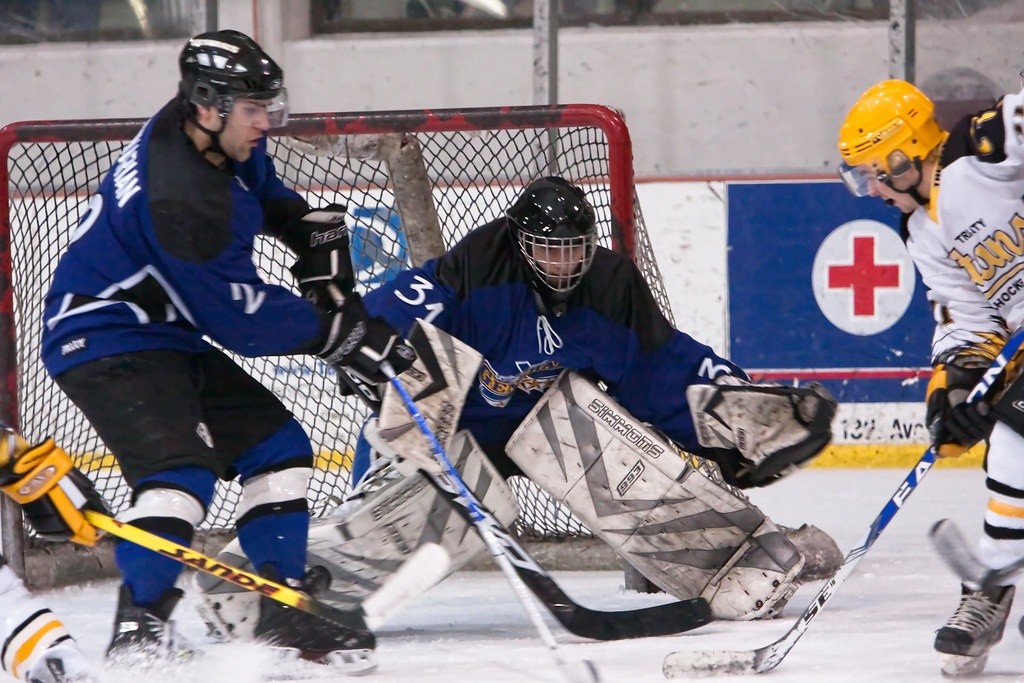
[194,177,835,645]
[0,427,115,683]
[39,31,420,671]
[839,79,1024,679]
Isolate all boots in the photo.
[105,583,194,666]
[253,562,379,675]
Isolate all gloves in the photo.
[1,437,114,547]
[924,362,1006,458]
[316,291,417,385]
[287,204,354,309]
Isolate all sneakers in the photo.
[934,581,1015,678]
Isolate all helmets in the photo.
[180,30,289,131]
[838,79,943,197]
[507,176,596,301]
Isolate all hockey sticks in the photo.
[334,364,712,640]
[327,280,616,683]
[84,510,454,639]
[662,326,1024,677]
